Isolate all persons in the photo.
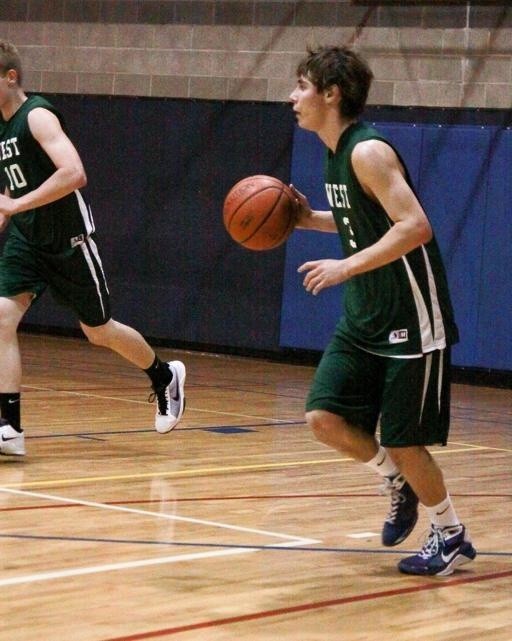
[287,44,476,576]
[1,40,188,457]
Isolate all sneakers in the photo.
[398,522,478,577]
[154,360,187,434]
[0,425,26,456]
[381,471,419,548]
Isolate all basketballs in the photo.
[223,174,297,250]
